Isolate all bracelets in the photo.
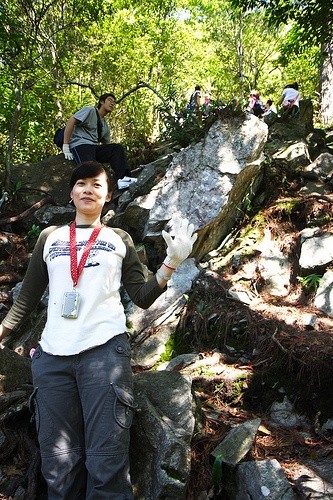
[161,261,177,270]
[157,269,171,280]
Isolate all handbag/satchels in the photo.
[54,127,65,150]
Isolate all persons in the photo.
[63,94,139,190]
[1,161,198,500]
[184,82,301,121]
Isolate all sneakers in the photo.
[117,176,138,189]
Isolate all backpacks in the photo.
[252,98,264,118]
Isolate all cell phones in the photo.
[61,292,80,319]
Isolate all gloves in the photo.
[62,144,75,160]
[161,218,198,270]
